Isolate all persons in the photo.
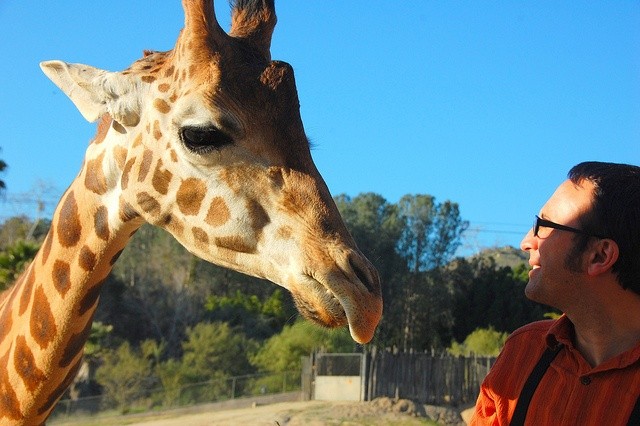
[468,161,639,425]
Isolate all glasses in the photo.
[534,216,599,236]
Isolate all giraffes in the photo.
[0,0,381,425]
